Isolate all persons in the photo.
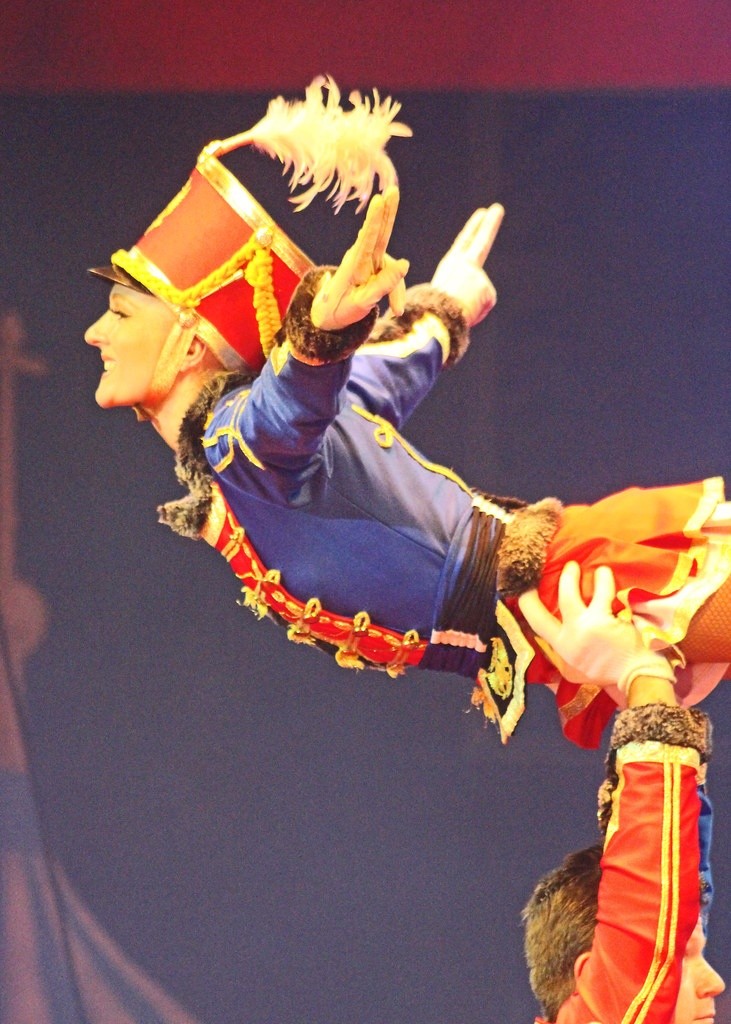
[518,560,730,1024]
[82,68,730,744]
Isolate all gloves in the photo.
[515,560,678,702]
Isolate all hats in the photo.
[87,149,316,381]
